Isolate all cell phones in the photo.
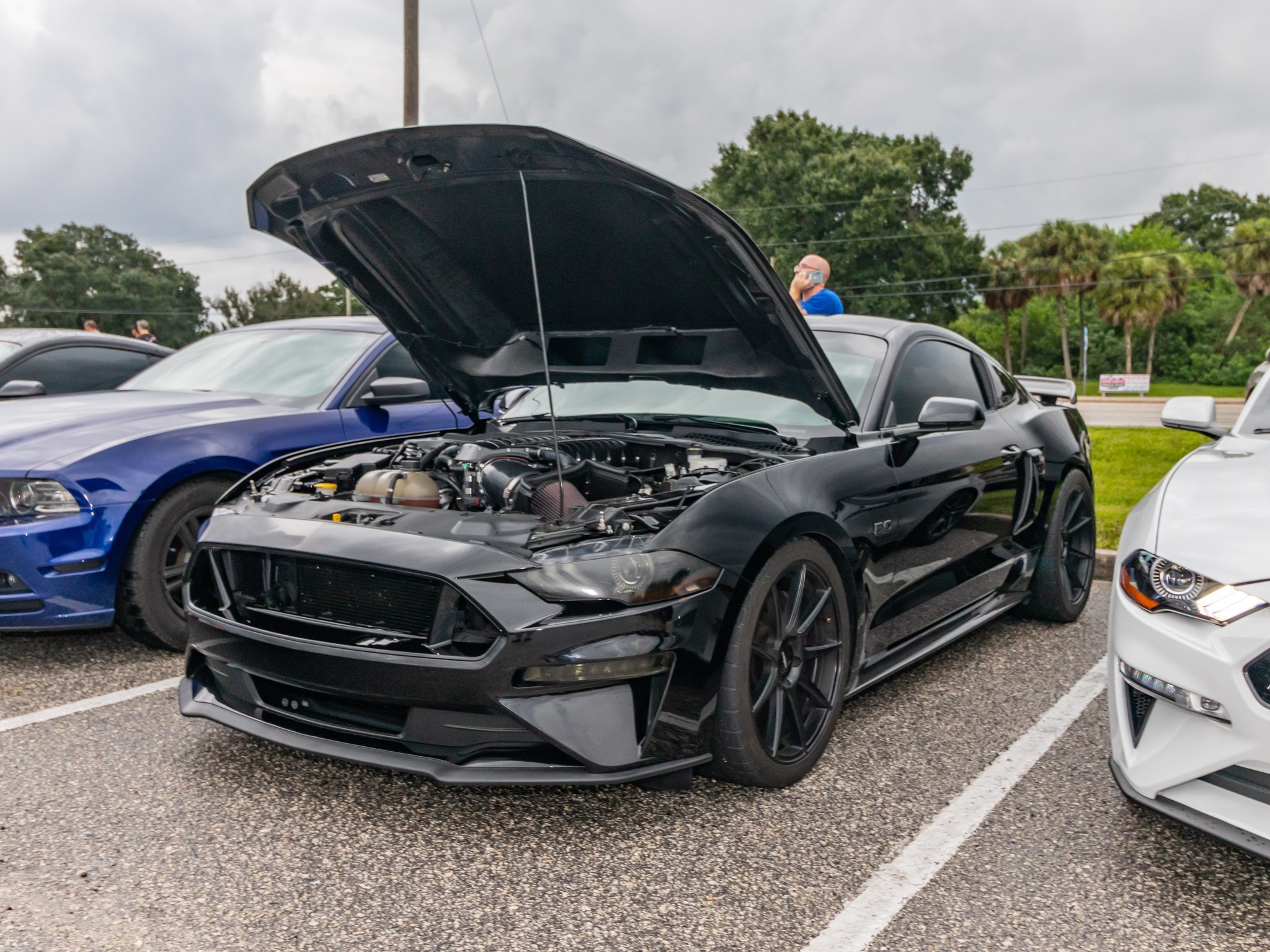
[806,270,824,286]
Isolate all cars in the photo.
[0,316,514,657]
[0,326,179,401]
[175,119,1098,805]
[1102,360,1270,859]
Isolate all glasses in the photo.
[792,264,823,275]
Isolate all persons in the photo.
[84,320,101,334]
[136,319,159,344]
[787,253,845,316]
[130,325,142,339]
